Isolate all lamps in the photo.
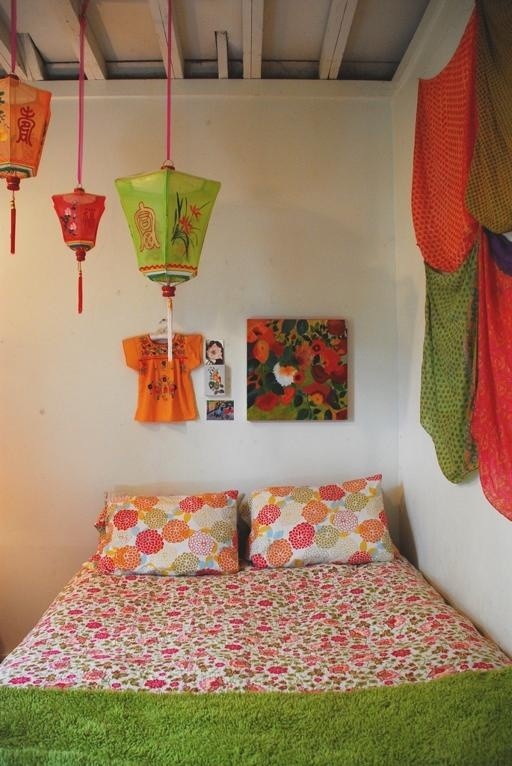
[0,0,52,254]
[114,0,221,362]
[52,0,106,314]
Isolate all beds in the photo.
[0,475,512,766]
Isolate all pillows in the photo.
[94,488,245,576]
[240,475,400,568]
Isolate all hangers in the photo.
[148,319,175,341]
